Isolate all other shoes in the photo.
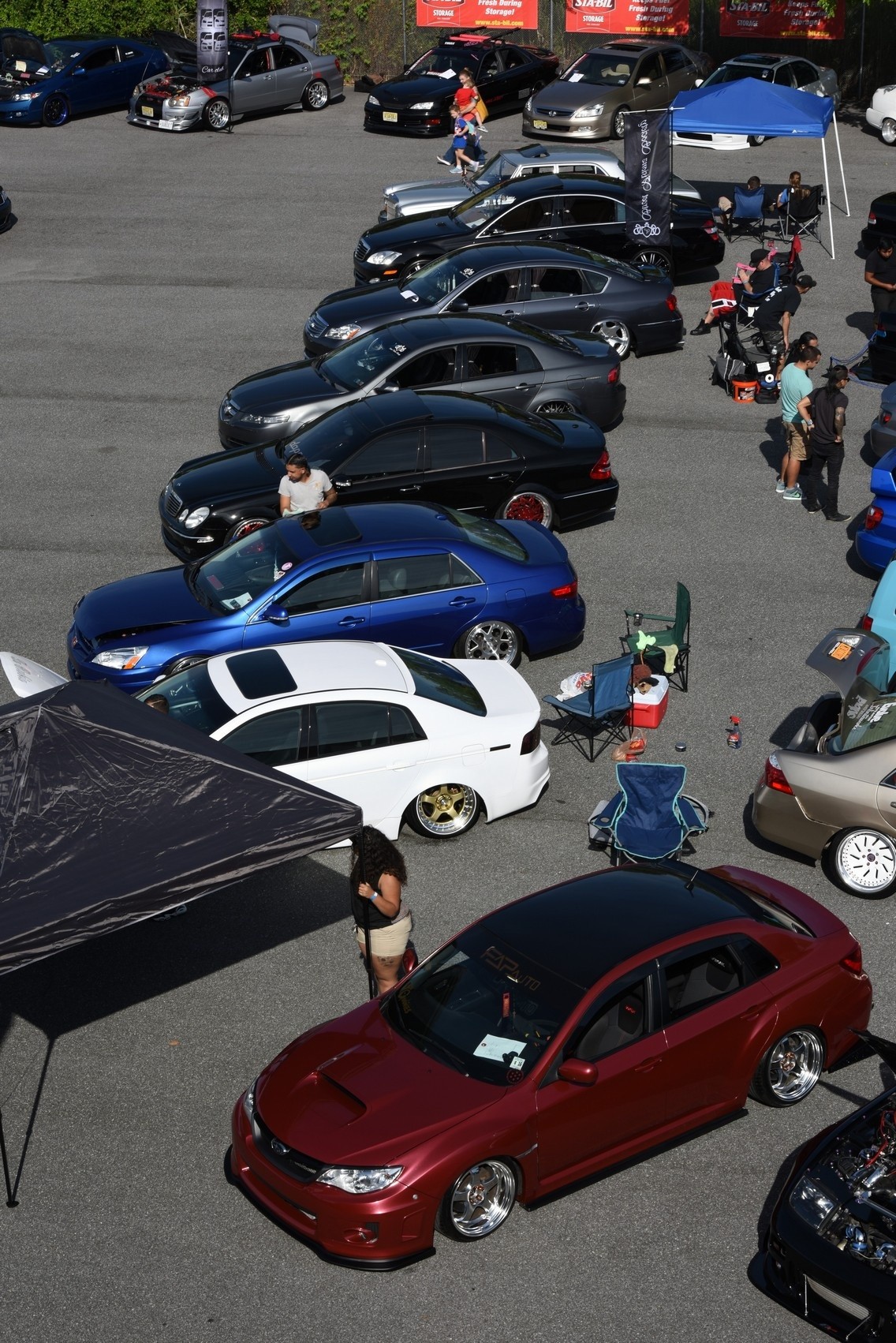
[469,164,484,167]
[437,157,451,166]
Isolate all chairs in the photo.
[769,233,803,282]
[733,265,779,334]
[623,581,692,693]
[721,187,766,243]
[775,183,821,244]
[593,759,705,862]
[542,653,638,763]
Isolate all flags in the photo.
[624,111,671,247]
[196,0,229,81]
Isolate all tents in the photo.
[658,77,851,260]
[0,681,380,1207]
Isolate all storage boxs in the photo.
[618,674,667,730]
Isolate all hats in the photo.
[797,275,817,287]
[749,248,771,268]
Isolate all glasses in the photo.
[841,378,851,382]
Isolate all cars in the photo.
[157,146,728,564]
[767,1027,896,1343]
[657,50,843,151]
[129,641,556,840]
[123,14,344,131]
[757,689,896,899]
[0,26,171,127]
[866,83,896,144]
[67,502,586,701]
[522,37,715,143]
[843,401,896,693]
[228,861,873,1273]
[361,24,561,139]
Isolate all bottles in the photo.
[770,346,777,364]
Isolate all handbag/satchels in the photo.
[472,89,489,123]
[612,726,648,761]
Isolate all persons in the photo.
[777,171,810,234]
[449,104,479,173]
[776,346,822,501]
[348,825,415,997]
[140,694,187,924]
[278,454,338,517]
[784,331,819,378]
[454,78,489,133]
[865,237,896,321]
[437,67,486,168]
[690,250,776,335]
[754,275,817,389]
[797,365,850,521]
[718,176,774,233]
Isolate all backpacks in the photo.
[457,119,477,145]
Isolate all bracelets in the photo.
[370,891,378,900]
[807,419,814,425]
[743,280,749,284]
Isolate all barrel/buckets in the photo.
[732,380,757,403]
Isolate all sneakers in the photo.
[783,486,807,500]
[690,318,712,335]
[776,480,801,493]
[469,160,479,172]
[808,503,825,514]
[478,125,488,132]
[826,512,851,522]
[450,167,464,173]
